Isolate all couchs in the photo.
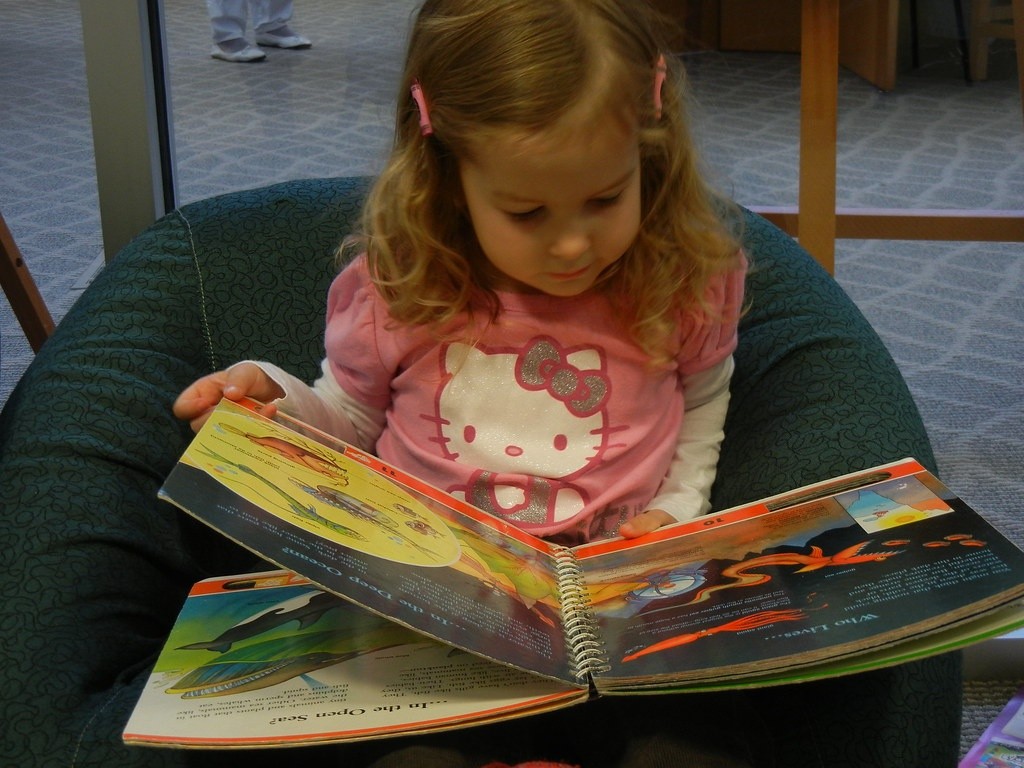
[0,175,965,768]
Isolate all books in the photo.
[123,394,1024,750]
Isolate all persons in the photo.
[175,0,745,768]
[207,0,312,61]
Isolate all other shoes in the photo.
[255,32,312,48]
[211,44,266,62]
